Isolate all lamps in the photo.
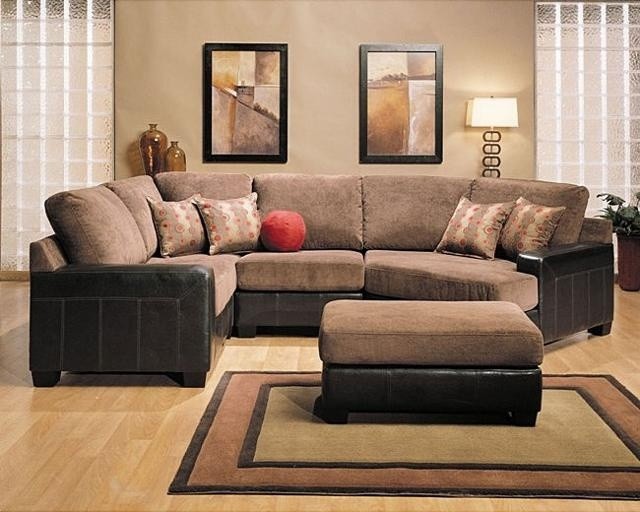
[461,94,521,176]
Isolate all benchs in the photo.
[316,300,544,429]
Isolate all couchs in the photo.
[21,172,613,389]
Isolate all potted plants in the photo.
[595,192,639,292]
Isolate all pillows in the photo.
[263,208,307,251]
[194,190,268,253]
[437,195,515,263]
[149,193,208,258]
[502,196,566,252]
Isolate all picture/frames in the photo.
[201,41,291,165]
[356,41,446,166]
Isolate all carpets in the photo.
[169,370,638,502]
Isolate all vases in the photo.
[140,121,169,172]
[161,138,185,171]
